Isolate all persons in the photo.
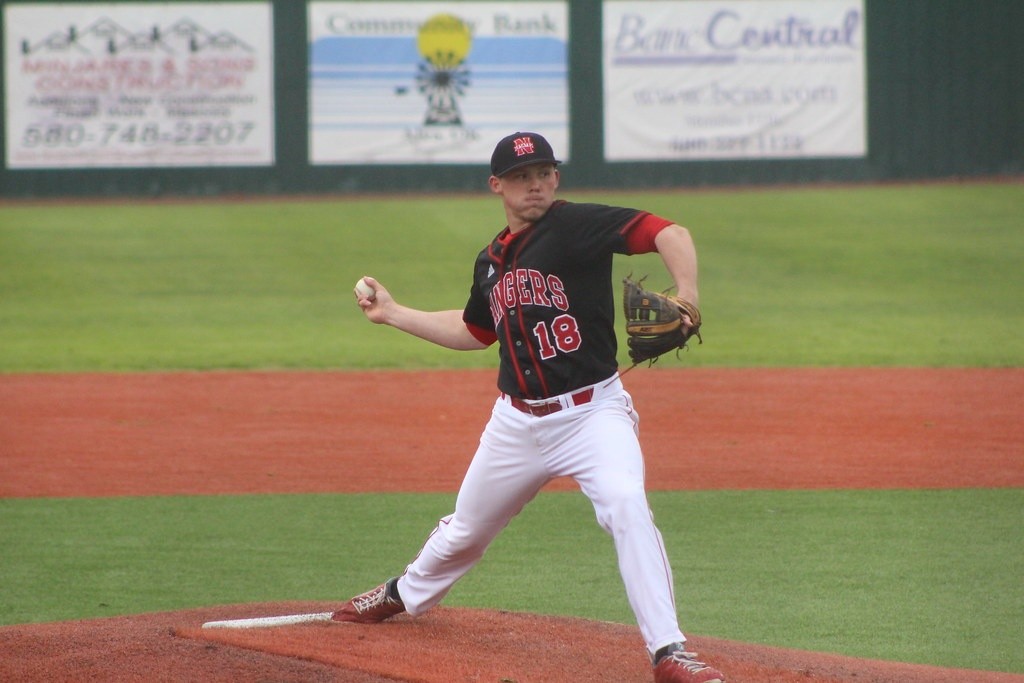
[334,131,722,683]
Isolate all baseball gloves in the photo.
[621,275,701,362]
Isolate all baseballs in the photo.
[355,278,376,302]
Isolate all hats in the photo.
[491,132,561,177]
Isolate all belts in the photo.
[502,389,593,416]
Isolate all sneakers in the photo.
[651,642,726,683]
[331,577,406,624]
